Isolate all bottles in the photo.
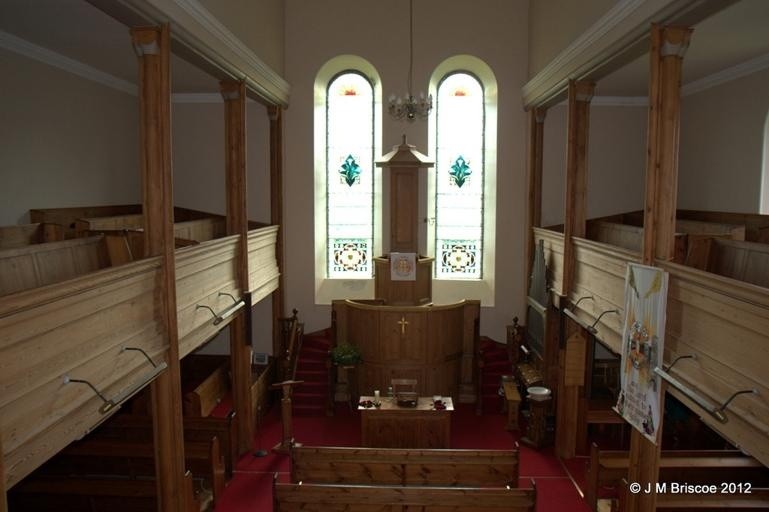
[387,387,392,402]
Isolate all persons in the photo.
[641,378,658,433]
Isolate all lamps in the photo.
[563,296,618,334]
[195,294,246,325]
[388,0,433,120]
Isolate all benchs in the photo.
[0,213,226,298]
[5,360,768,512]
[590,217,768,288]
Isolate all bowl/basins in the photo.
[528,386,551,401]
